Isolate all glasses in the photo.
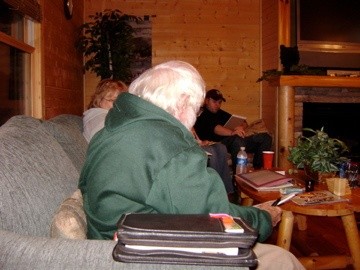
[212,99,222,104]
[190,103,203,117]
[103,97,116,104]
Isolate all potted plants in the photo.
[290,126,346,183]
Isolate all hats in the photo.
[206,89,226,102]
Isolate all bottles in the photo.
[236,147,248,174]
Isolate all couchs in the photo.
[0,115,306,270]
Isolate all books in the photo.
[225,115,246,129]
[235,168,295,192]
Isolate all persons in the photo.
[193,90,274,198]
[82,79,128,144]
[80,59,305,270]
[190,127,238,203]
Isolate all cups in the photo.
[262,151,275,169]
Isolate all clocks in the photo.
[63,0,74,20]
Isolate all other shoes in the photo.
[228,193,236,202]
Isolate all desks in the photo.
[276,74,360,168]
[240,165,360,270]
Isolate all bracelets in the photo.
[230,131,235,136]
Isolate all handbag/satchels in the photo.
[112,212,259,270]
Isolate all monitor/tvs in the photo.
[296,0,360,45]
